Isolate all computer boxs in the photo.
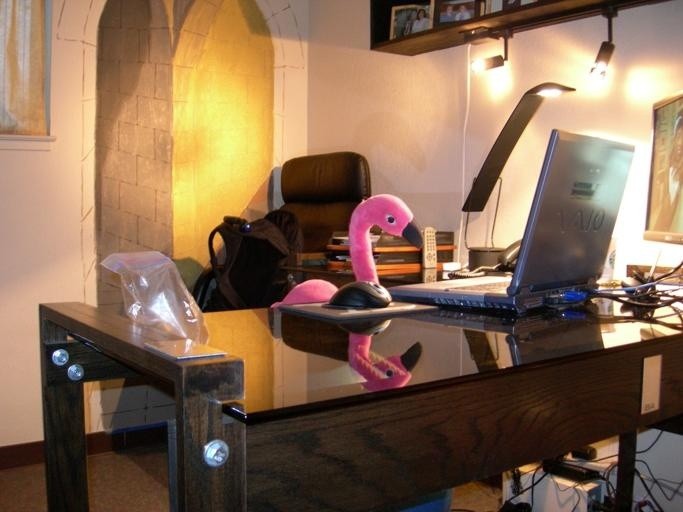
[502,462,601,512]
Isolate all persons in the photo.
[440,3,457,21]
[401,8,416,36]
[454,4,471,21]
[410,7,430,35]
[647,116,683,234]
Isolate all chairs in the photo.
[279,153,372,249]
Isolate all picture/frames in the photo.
[429,0,484,28]
[643,91,683,246]
[389,6,428,39]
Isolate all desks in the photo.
[38,283,683,512]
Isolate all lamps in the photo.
[483,24,514,70]
[461,81,578,213]
[594,9,619,68]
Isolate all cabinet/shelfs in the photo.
[370,0,667,56]
[284,266,442,291]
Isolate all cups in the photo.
[597,232,616,284]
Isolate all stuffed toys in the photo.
[346,330,424,393]
[264,192,425,308]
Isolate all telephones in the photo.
[498,239,523,271]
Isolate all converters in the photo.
[572,445,598,460]
[543,463,599,482]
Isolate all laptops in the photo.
[387,129,634,314]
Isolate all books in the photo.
[331,232,406,281]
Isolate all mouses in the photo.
[339,319,392,336]
[330,281,393,308]
